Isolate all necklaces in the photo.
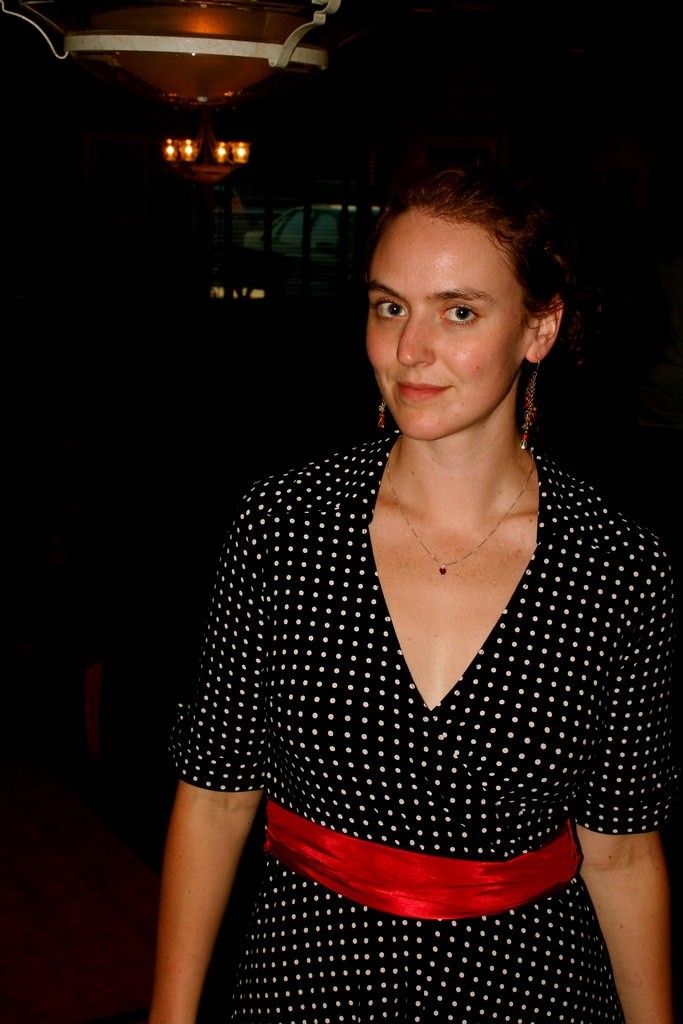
[385,450,534,574]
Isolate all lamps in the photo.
[0,0,343,186]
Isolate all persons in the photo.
[145,176,683,1024]
